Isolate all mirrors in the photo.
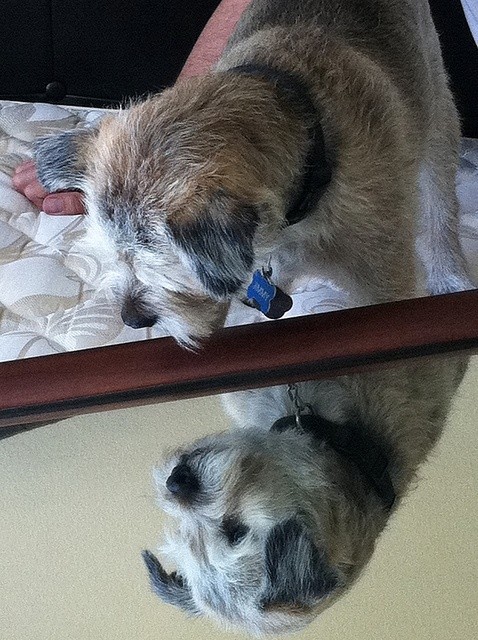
[0,288,478,640]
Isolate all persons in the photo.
[13,0,256,213]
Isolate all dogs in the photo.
[31,0,476,353]
[138,348,471,638]
